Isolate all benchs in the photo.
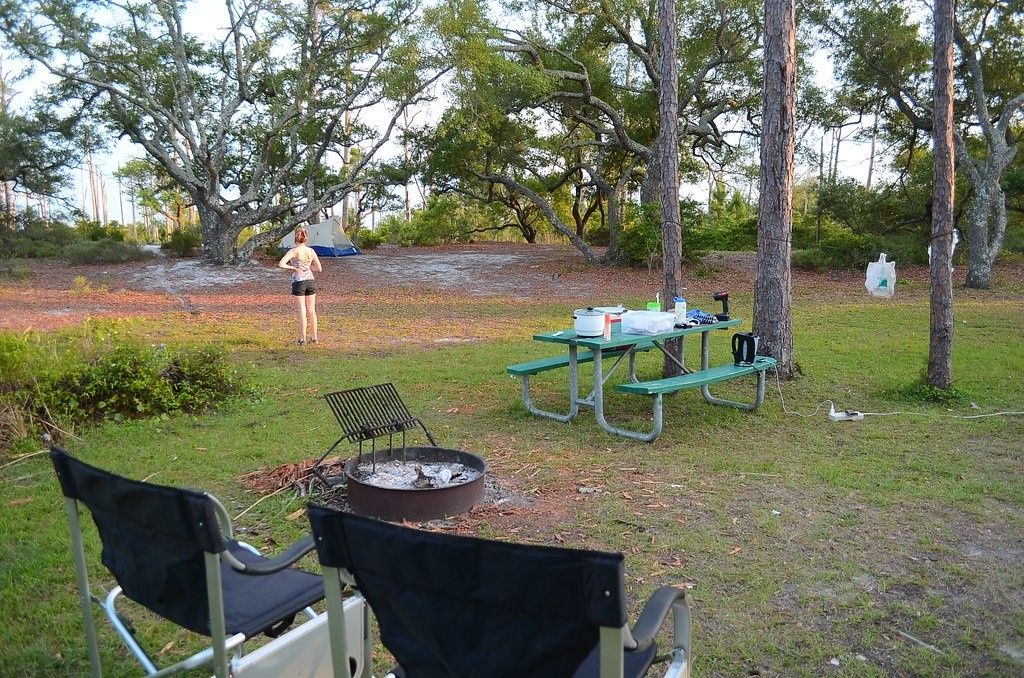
[506,342,666,409]
[615,356,778,436]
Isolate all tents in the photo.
[277,217,362,256]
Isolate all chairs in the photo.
[51,445,374,678]
[305,498,692,678]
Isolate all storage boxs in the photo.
[621,310,674,335]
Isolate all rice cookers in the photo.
[571,307,607,337]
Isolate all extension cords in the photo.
[828,411,864,422]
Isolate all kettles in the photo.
[732,332,760,366]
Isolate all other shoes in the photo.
[306,338,319,345]
[294,339,307,346]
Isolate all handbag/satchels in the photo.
[865,253,896,299]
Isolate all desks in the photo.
[532,319,743,441]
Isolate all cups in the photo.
[673,297,687,323]
[646,302,661,331]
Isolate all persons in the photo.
[278,228,322,345]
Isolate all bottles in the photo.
[604,313,611,341]
